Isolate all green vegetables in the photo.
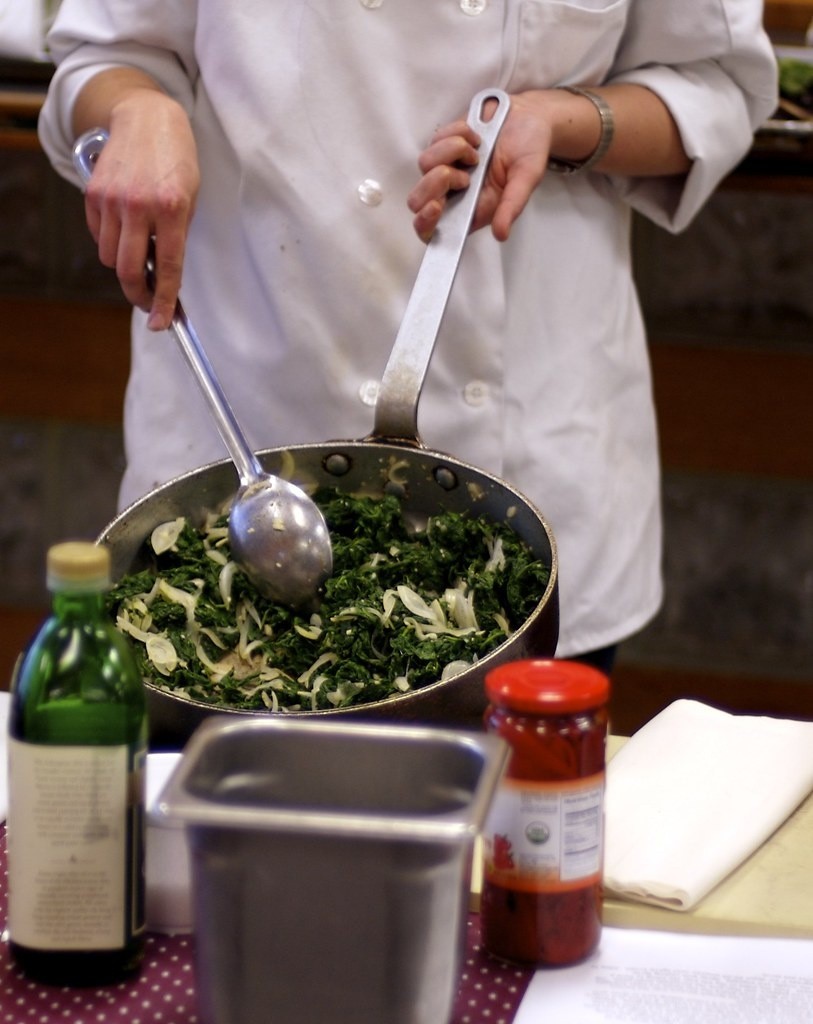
[106,484,551,712]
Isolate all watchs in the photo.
[548,86,614,178]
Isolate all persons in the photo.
[36,0,780,676]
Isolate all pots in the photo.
[95,86,559,758]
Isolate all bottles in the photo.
[484,660,611,971]
[6,542,150,988]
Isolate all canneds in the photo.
[482,659,612,966]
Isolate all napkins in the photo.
[597,698,813,910]
